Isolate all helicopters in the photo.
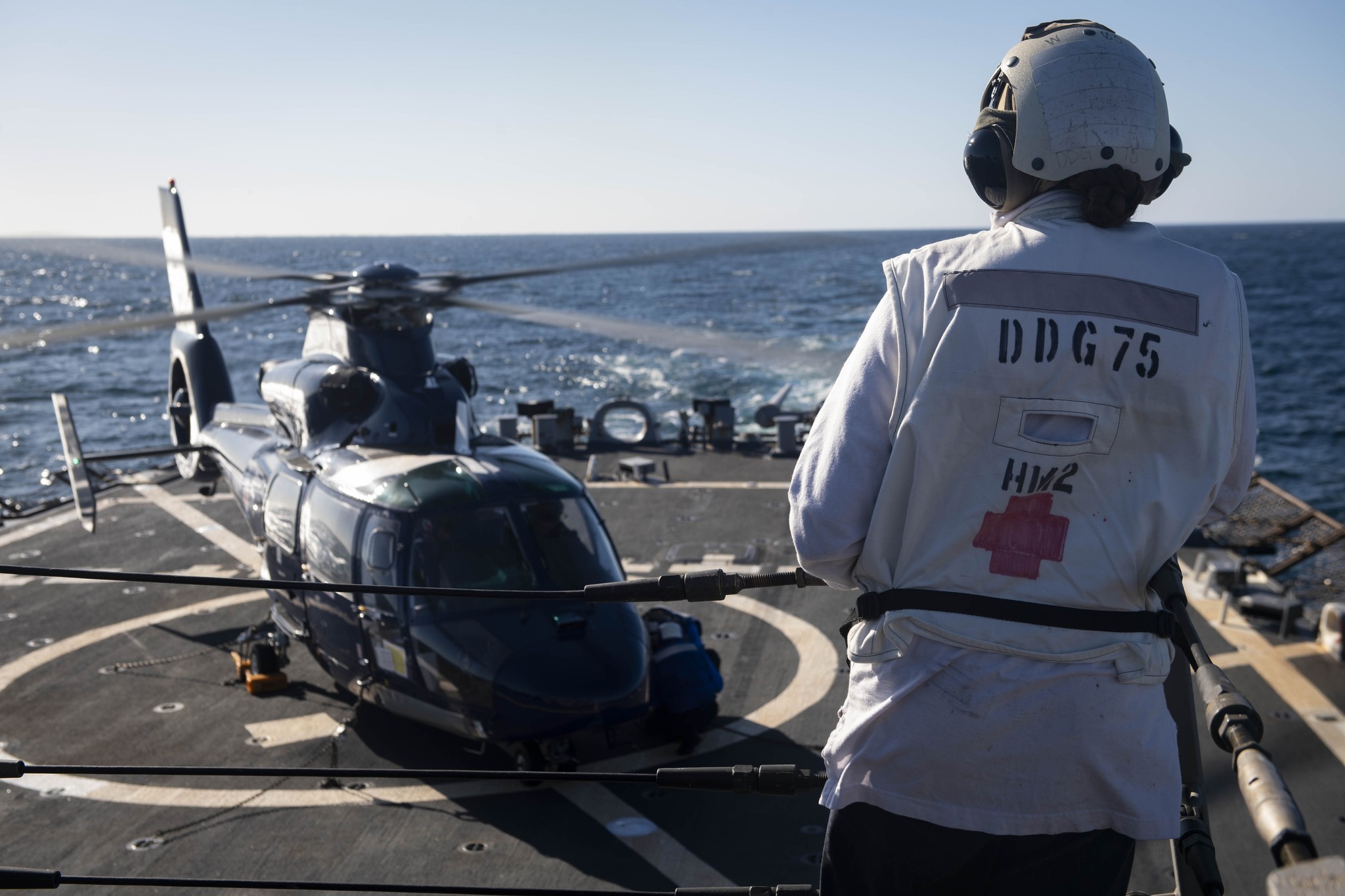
[0,179,891,747]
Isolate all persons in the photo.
[790,11,1260,896]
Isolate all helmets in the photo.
[965,18,1191,225]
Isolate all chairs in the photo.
[1188,488,1344,641]
